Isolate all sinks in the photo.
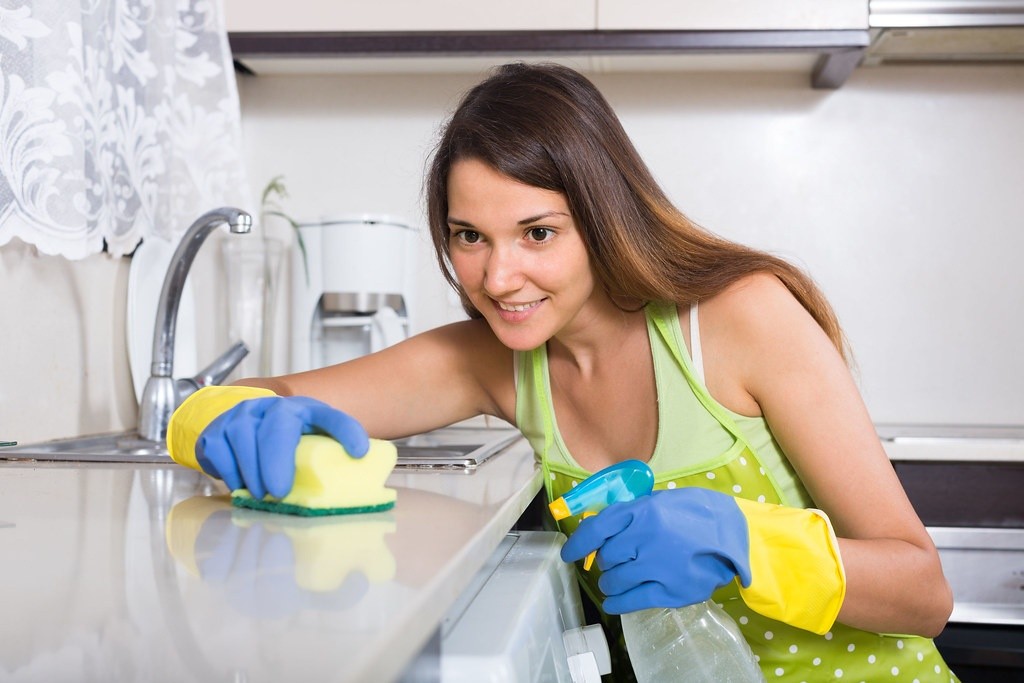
[0,426,521,473]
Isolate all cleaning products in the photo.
[546,458,765,683]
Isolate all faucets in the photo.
[137,204,253,446]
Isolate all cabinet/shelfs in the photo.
[214,1,872,77]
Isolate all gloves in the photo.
[560,487,846,634]
[166,386,369,501]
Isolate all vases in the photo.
[221,234,297,383]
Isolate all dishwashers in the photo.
[443,532,612,683]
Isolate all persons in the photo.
[166,62,959,683]
